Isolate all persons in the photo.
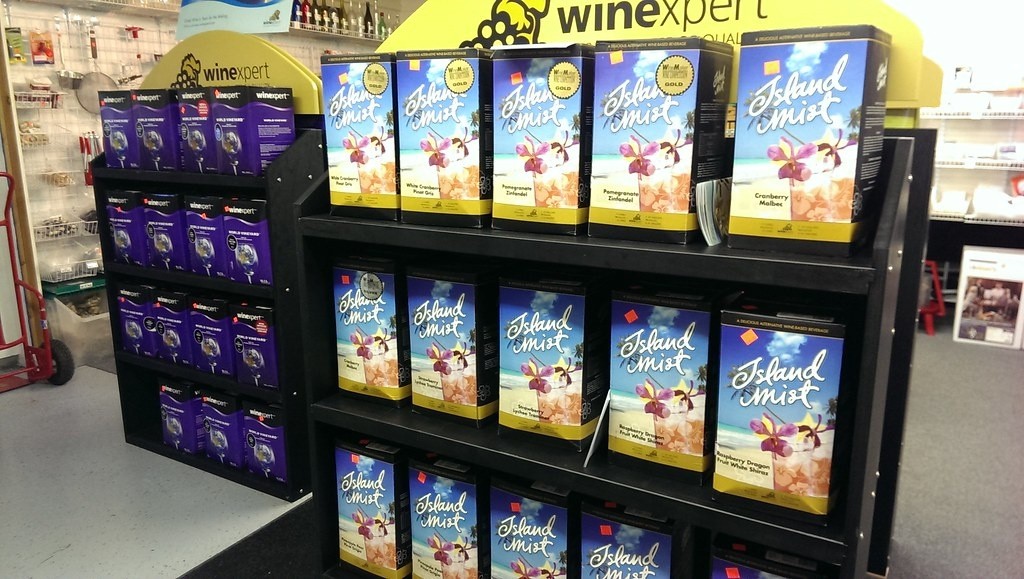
[964,278,1008,317]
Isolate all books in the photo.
[696,177,732,247]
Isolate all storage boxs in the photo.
[96,84,294,486]
[951,245,1024,350]
[321,24,891,578]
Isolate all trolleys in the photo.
[0,171,75,393]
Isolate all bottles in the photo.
[291,0,401,40]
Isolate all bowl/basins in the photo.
[56,70,85,89]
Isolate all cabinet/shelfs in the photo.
[86,129,326,504]
[917,87,1024,229]
[285,132,916,578]
